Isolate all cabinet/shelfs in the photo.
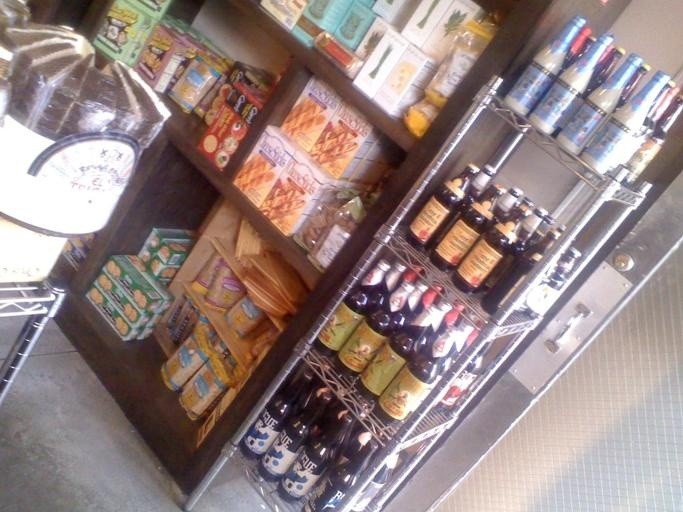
[52,0,681,512]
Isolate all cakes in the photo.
[2,20,174,153]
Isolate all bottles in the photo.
[239,345,403,511]
[503,11,683,189]
[315,252,495,427]
[402,162,580,322]
